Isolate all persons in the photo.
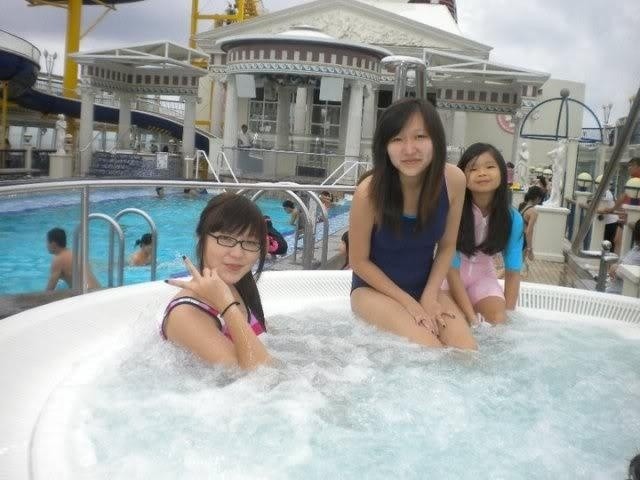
[506,162,514,185]
[155,187,166,198]
[178,187,200,198]
[129,232,154,264]
[153,191,276,373]
[44,227,103,290]
[519,185,544,273]
[604,219,640,294]
[338,231,353,270]
[440,141,524,326]
[597,156,639,252]
[316,191,330,223]
[263,216,288,259]
[348,95,477,355]
[605,189,620,252]
[236,124,254,176]
[281,200,305,230]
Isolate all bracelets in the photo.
[220,300,241,317]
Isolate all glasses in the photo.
[207,233,261,251]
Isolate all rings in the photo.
[420,318,426,323]
